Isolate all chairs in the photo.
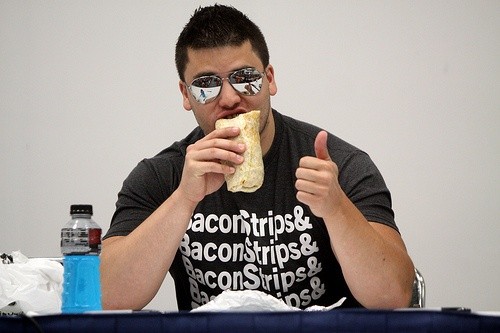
[414,268,425,309]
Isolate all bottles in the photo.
[60,204,102,313]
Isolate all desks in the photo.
[32,308,500,332]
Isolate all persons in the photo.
[99,4,415,310]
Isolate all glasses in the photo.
[183,67,265,105]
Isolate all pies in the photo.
[214,110,265,193]
